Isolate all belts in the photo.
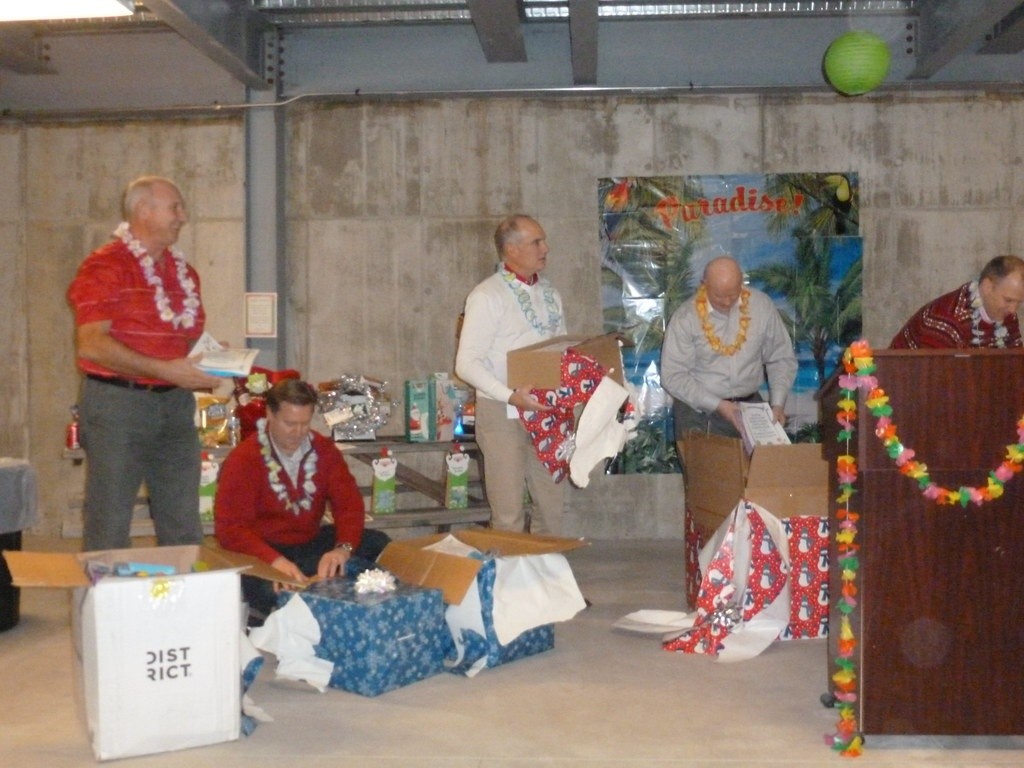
[86,373,178,393]
[725,392,756,403]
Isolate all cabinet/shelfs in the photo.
[62,436,532,544]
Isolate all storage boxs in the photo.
[2,545,306,761]
[671,431,830,642]
[377,529,592,679]
[506,334,636,410]
[276,574,446,697]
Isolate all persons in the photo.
[454,214,567,536]
[66,178,229,553]
[888,255,1024,350]
[660,257,799,467]
[214,378,391,618]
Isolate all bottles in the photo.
[228,407,241,447]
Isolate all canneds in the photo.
[67,422,80,449]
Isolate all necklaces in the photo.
[497,265,561,335]
[696,284,751,356]
[256,417,318,515]
[112,221,201,329]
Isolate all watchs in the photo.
[337,542,354,552]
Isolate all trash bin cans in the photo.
[0,458,28,633]
